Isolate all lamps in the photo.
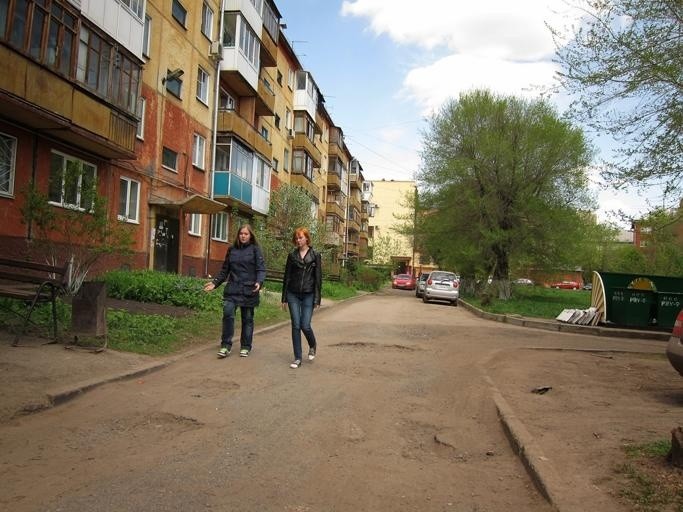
[162,67,184,86]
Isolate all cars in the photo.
[662,308,683,378]
[475,277,592,290]
[390,270,462,306]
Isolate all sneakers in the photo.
[291,359,301,368]
[308,348,316,361]
[241,350,249,357]
[218,348,231,357]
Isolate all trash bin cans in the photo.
[64,281,107,352]
[591,271,682,328]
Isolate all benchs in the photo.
[0,256,69,348]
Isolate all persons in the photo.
[203,223,267,357]
[281,226,322,369]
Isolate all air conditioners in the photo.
[287,128,295,140]
[211,41,223,58]
